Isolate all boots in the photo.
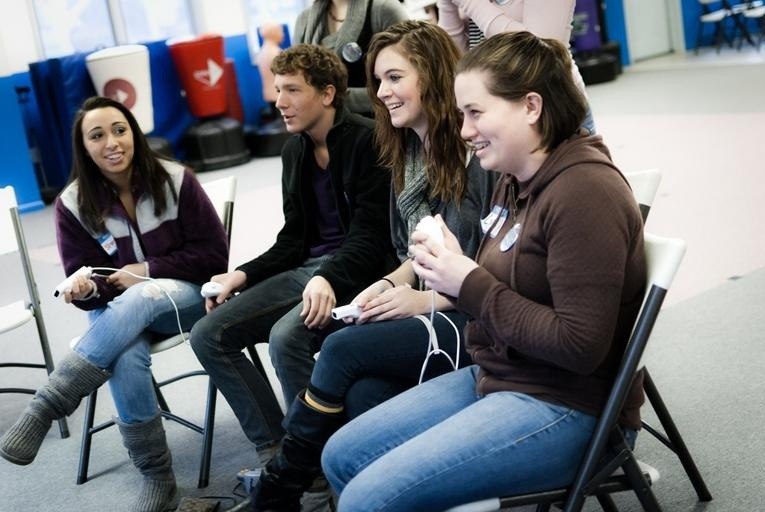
[111,408,176,511]
[226,388,347,511]
[0,350,111,466]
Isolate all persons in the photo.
[0,96,229,512]
[188,41,401,510]
[291,0,412,122]
[226,20,504,512]
[435,0,596,135]
[253,18,285,118]
[320,29,648,511]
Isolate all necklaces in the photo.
[328,8,344,22]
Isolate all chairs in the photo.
[589,171,660,238]
[0,182,71,441]
[499,241,713,510]
[75,178,238,484]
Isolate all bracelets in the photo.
[380,276,396,289]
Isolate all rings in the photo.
[419,269,426,281]
